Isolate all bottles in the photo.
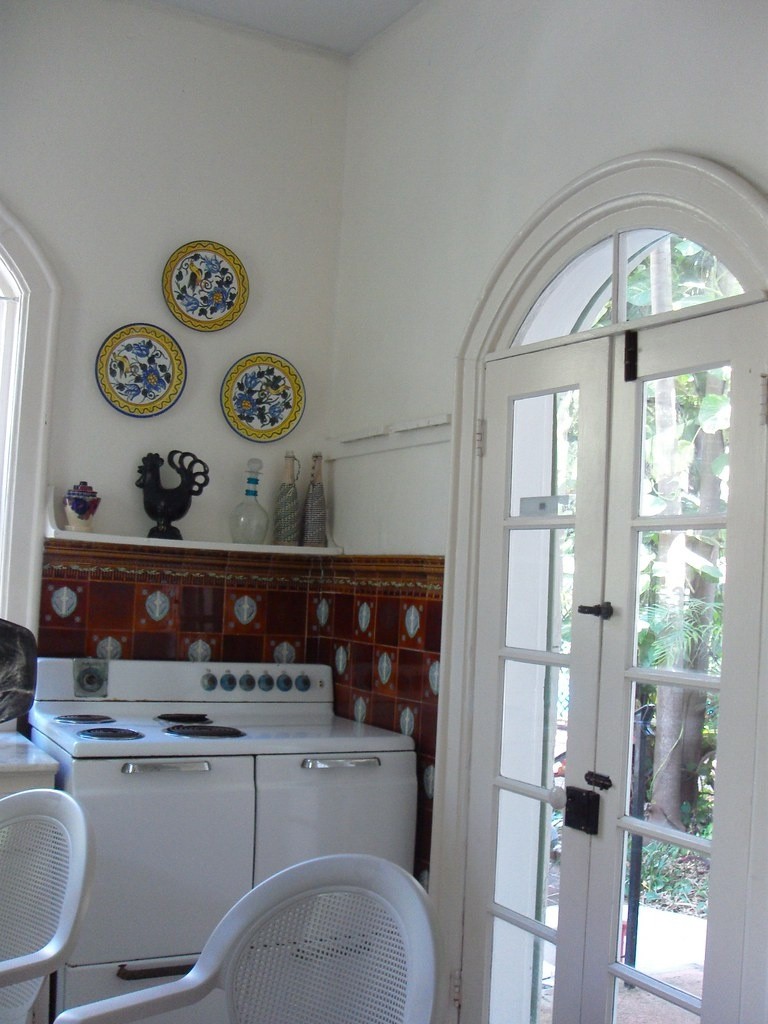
[230,458,270,545]
[273,448,300,547]
[301,450,329,548]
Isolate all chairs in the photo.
[0,788,89,1023]
[45,852,443,1024]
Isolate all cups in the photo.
[61,481,102,527]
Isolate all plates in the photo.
[95,324,187,419]
[162,239,249,334]
[219,351,306,444]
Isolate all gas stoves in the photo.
[29,654,416,760]
